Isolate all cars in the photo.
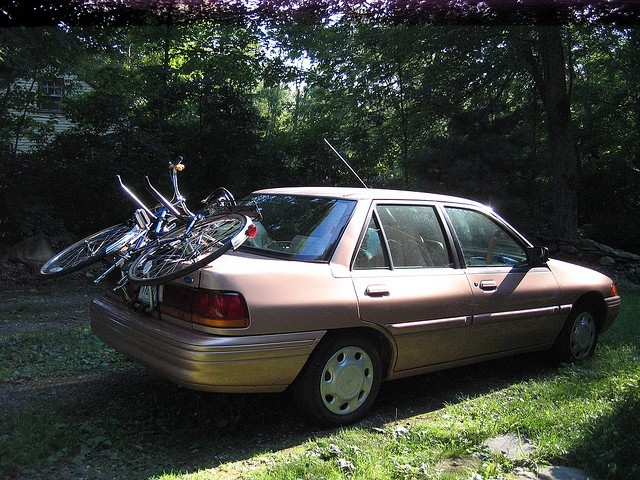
[91,186,620,425]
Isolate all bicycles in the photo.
[41,157,257,286]
